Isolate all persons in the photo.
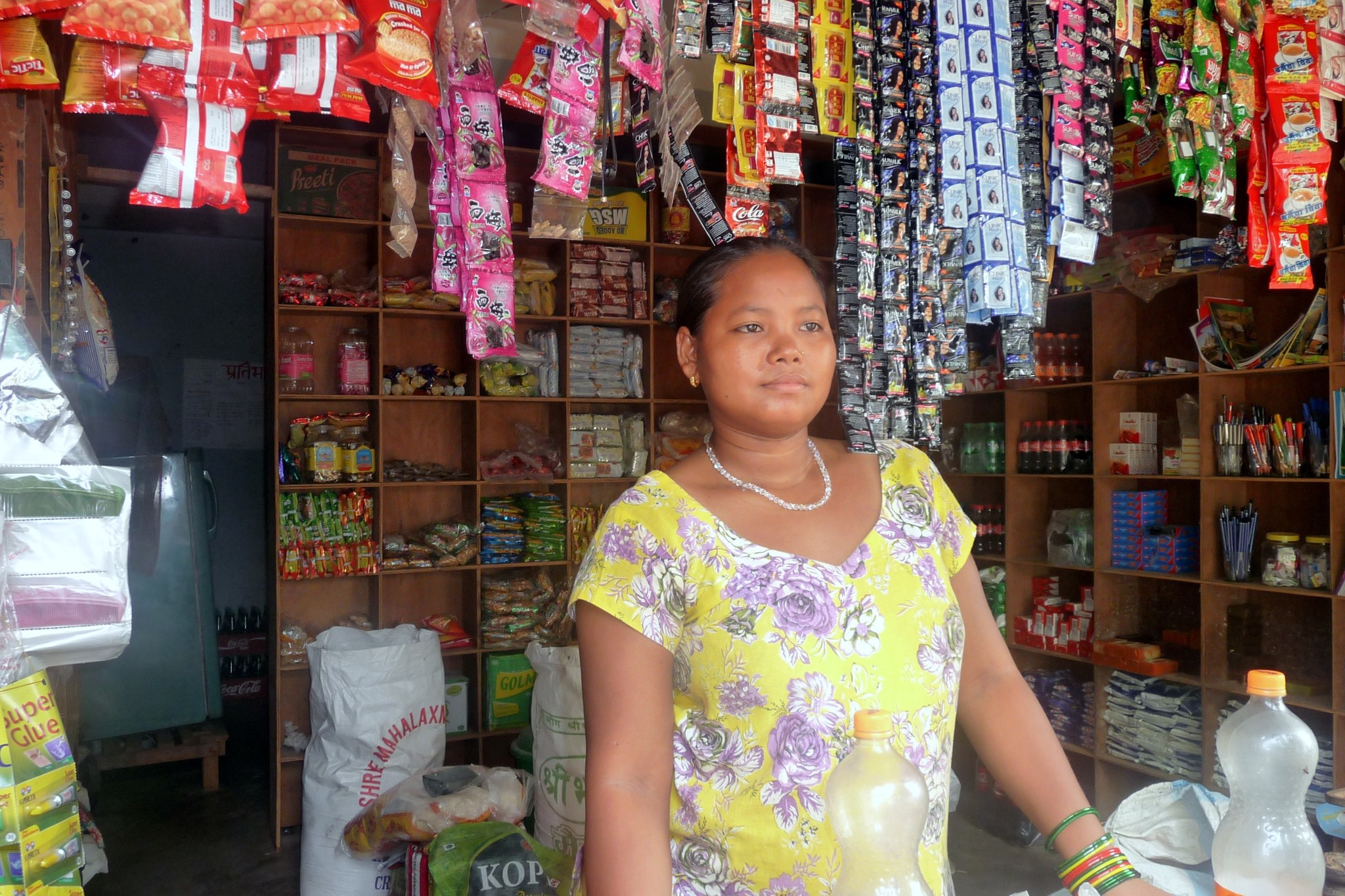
[561,235,1176,895]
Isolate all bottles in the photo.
[1260,532,1333,593]
[276,323,315,395]
[1211,668,1327,895]
[957,335,1093,564]
[335,328,373,395]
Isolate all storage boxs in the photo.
[1109,112,1173,191]
[277,142,380,221]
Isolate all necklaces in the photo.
[703,431,832,510]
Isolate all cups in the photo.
[1216,443,1330,477]
[1224,552,1252,583]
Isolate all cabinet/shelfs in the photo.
[916,104,1345,855]
[273,124,848,853]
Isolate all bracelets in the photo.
[1056,832,1142,895]
[1044,806,1101,855]
